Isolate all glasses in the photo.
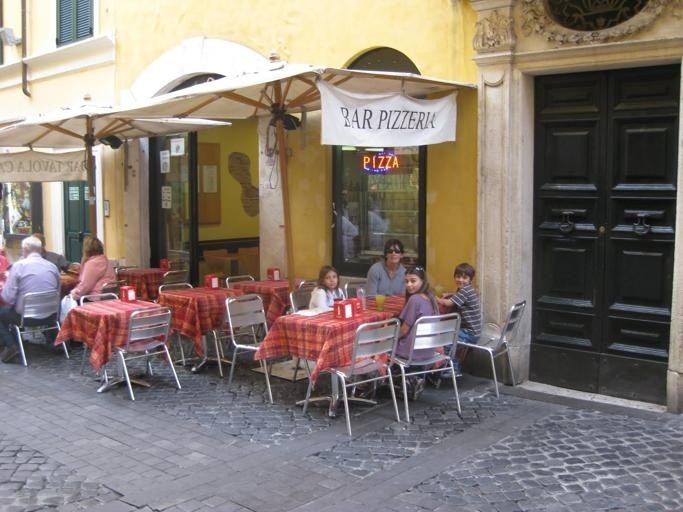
[385,249,401,253]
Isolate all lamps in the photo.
[97,135,128,149]
[0,26,23,47]
[269,111,303,131]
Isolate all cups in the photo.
[435,286,443,299]
[374,290,385,311]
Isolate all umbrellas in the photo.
[109,50,478,373]
[0,96,231,237]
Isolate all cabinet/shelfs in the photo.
[180,141,221,226]
[358,207,420,261]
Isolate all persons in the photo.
[388,264,440,401]
[365,240,409,297]
[310,266,345,310]
[336,188,383,259]
[19,233,79,274]
[1,238,61,363]
[1,252,12,281]
[435,264,482,378]
[60,237,116,325]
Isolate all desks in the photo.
[76,298,162,394]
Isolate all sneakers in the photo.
[442,369,461,378]
[410,376,425,400]
[3,344,20,363]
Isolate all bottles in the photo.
[356,285,367,311]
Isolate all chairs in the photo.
[101,308,183,401]
[3,258,318,378]
[431,299,526,399]
[76,293,126,384]
[288,291,319,388]
[310,317,399,435]
[382,313,461,422]
[225,294,314,404]
